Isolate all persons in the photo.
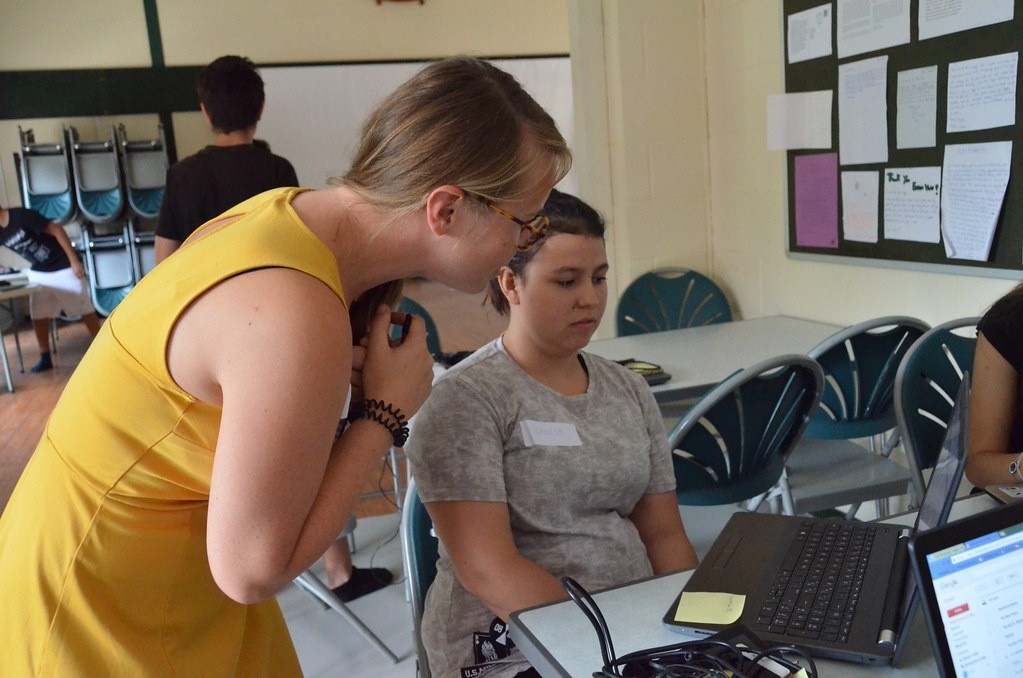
[1,53,574,677]
[963,277,1023,490]
[398,185,703,678]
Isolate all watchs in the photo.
[1008,452,1023,484]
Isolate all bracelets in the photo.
[350,396,410,449]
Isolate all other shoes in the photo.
[32,359,53,373]
[328,565,394,603]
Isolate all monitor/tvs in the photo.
[906,496,1023,678]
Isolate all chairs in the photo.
[669,316,983,518]
[17,122,169,319]
[401,476,439,678]
[615,268,733,336]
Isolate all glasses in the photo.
[463,191,549,250]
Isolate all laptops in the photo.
[661,369,971,669]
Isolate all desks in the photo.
[508,491,1000,678]
[0,286,60,393]
[344,315,857,602]
